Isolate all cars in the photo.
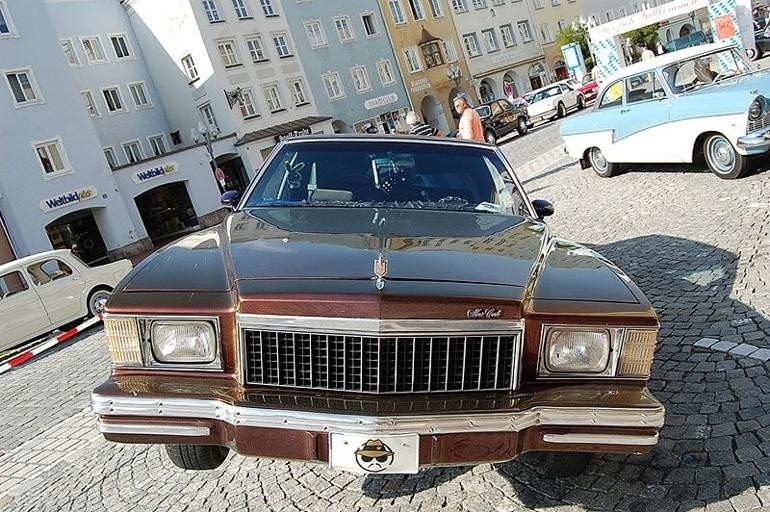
[1,249,133,354]
[561,44,769,179]
[745,19,770,61]
[473,73,598,145]
[91,132,666,479]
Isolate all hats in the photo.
[405,110,421,125]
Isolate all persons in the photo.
[405,110,445,138]
[558,70,563,80]
[651,58,725,98]
[641,45,655,60]
[452,96,486,143]
[591,62,604,86]
[655,40,668,55]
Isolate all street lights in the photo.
[571,16,595,64]
[446,64,462,88]
[190,122,227,191]
